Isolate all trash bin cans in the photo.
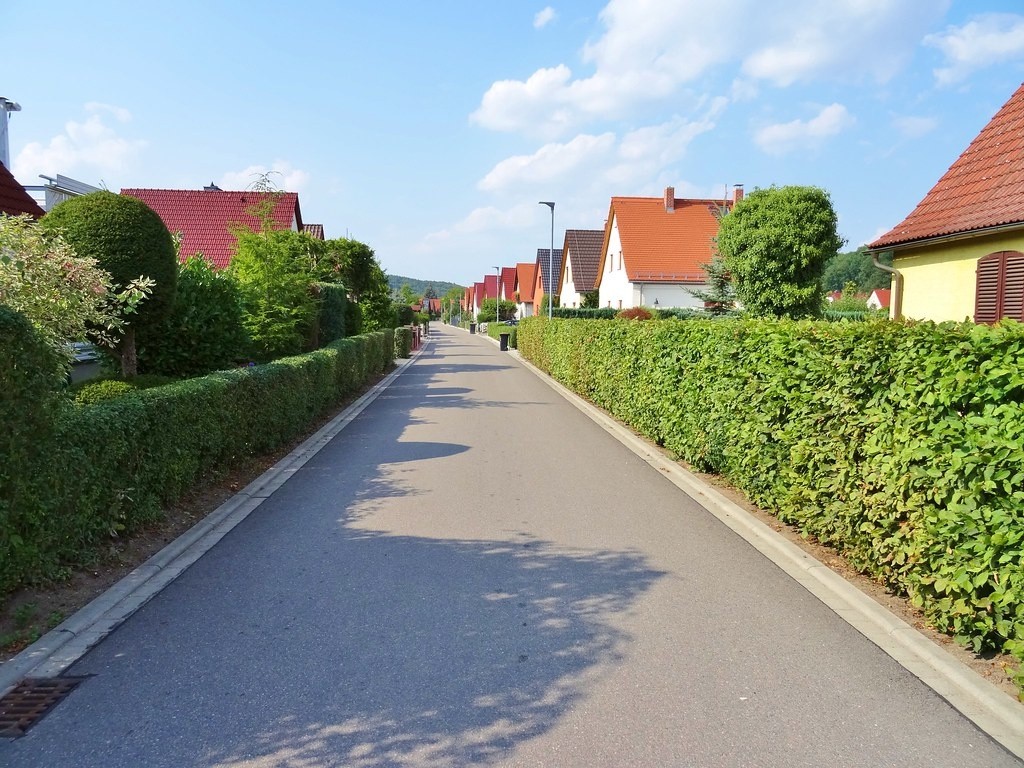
[500,334,510,351]
[470,324,477,334]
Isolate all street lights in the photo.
[538,201,555,322]
[458,292,461,322]
[493,266,499,324]
[440,298,451,325]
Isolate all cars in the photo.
[504,320,519,326]
